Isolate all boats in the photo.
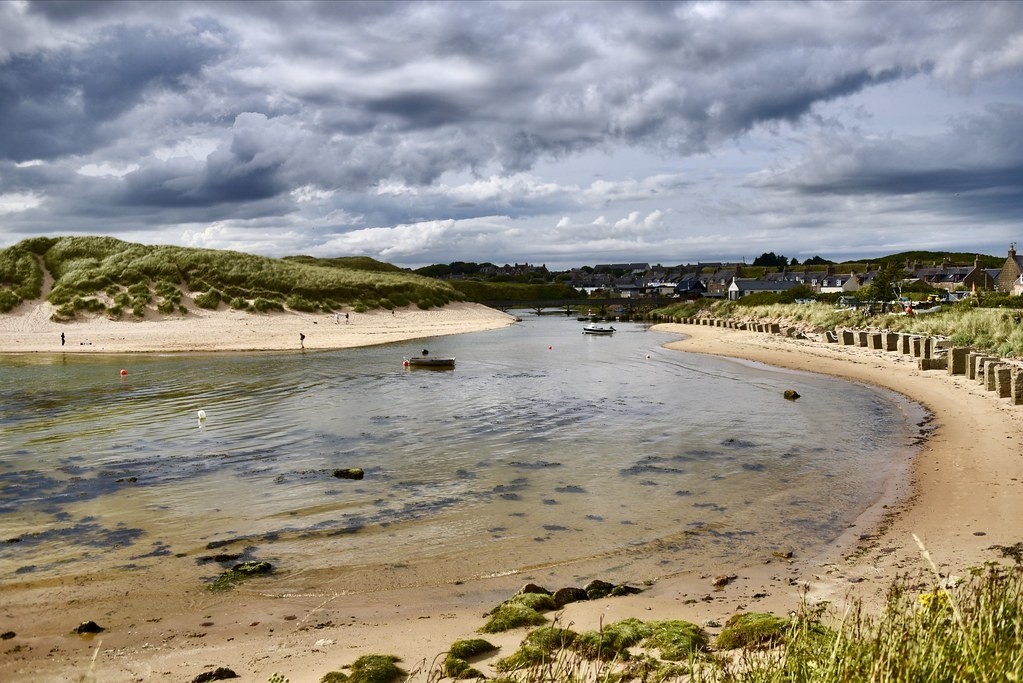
[583,323,614,335]
[409,357,457,365]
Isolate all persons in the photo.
[60,332,66,346]
[333,312,350,324]
[390,309,397,316]
[298,332,306,349]
